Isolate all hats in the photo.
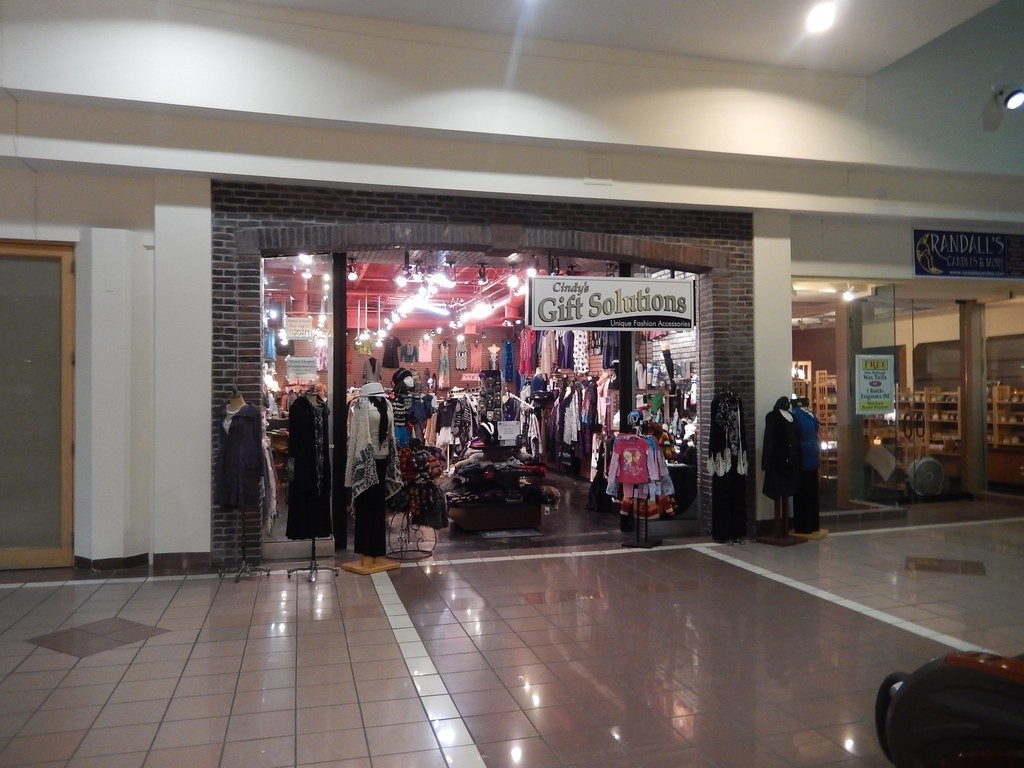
[392,367,412,383]
[355,382,388,397]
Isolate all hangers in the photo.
[633,426,651,438]
[449,387,480,397]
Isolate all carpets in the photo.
[478,529,543,539]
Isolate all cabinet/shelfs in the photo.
[794,360,1024,484]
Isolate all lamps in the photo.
[477,262,489,284]
[376,295,383,346]
[354,299,363,343]
[347,257,358,280]
[396,250,413,287]
[360,295,371,338]
[548,253,560,274]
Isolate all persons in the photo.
[286,385,333,540]
[792,397,819,533]
[216,390,264,508]
[521,367,547,431]
[762,397,804,536]
[391,368,414,445]
[345,383,404,558]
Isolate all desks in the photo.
[448,419,541,531]
